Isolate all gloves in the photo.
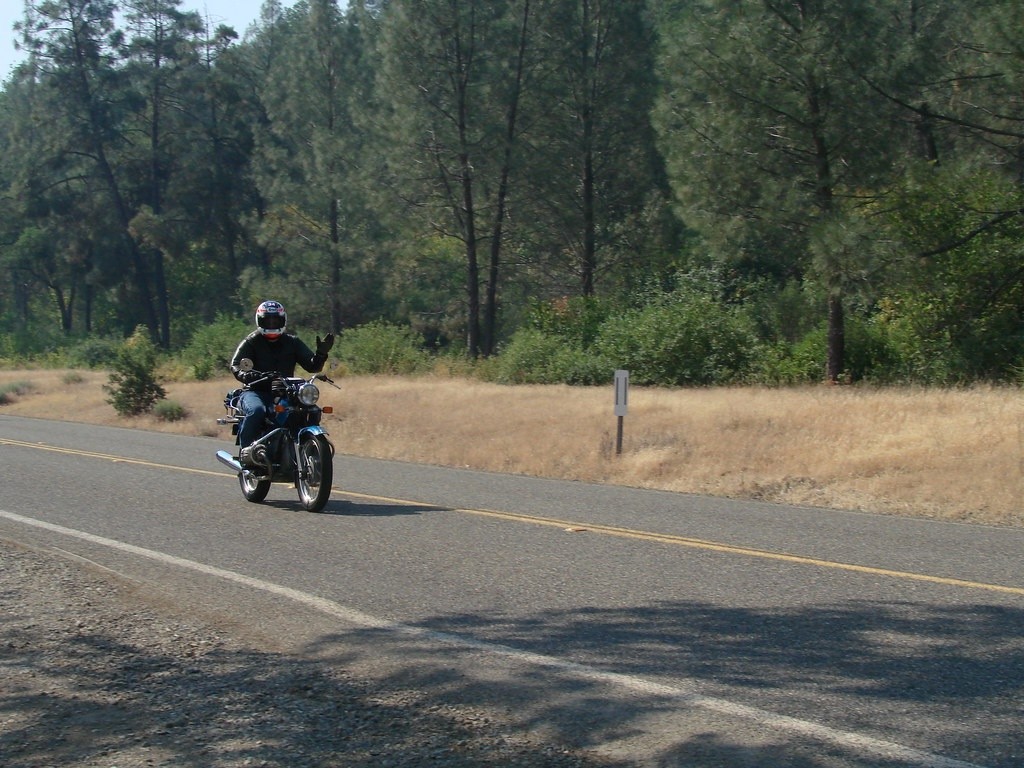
[245,372,260,383]
[316,333,334,355]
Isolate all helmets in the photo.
[256,301,287,339]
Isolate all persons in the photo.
[231,300,335,466]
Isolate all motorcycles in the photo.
[215,358,341,513]
